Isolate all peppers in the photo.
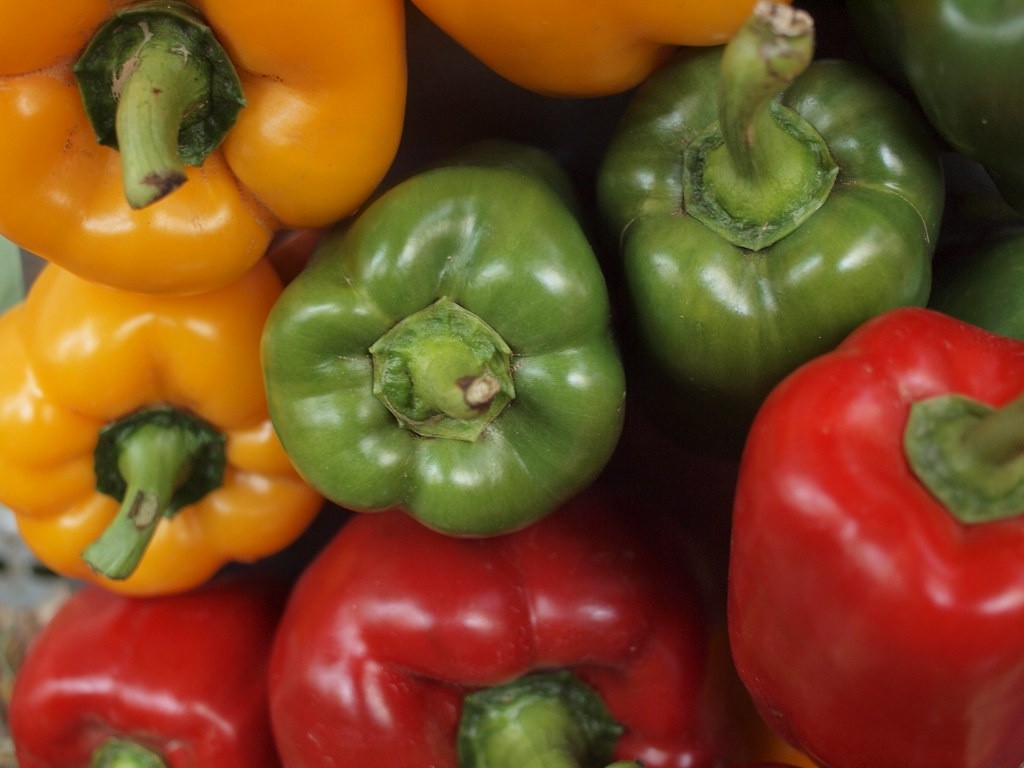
[0,0,1024,768]
